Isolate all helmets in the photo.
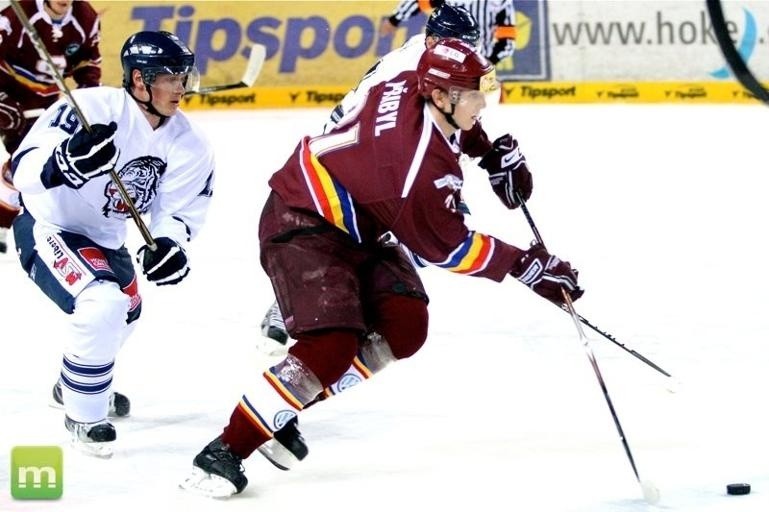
[426,5,480,43]
[121,30,194,87]
[417,37,496,98]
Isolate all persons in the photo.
[377,0,523,80]
[190,40,584,492]
[13,29,217,446]
[255,9,502,352]
[0,1,102,254]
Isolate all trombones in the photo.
[516,194,660,505]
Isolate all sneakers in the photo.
[262,299,287,344]
[65,414,116,442]
[193,438,247,494]
[52,379,129,417]
[274,415,308,461]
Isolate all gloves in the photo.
[510,243,584,302]
[137,237,190,286]
[478,133,531,210]
[53,122,121,190]
